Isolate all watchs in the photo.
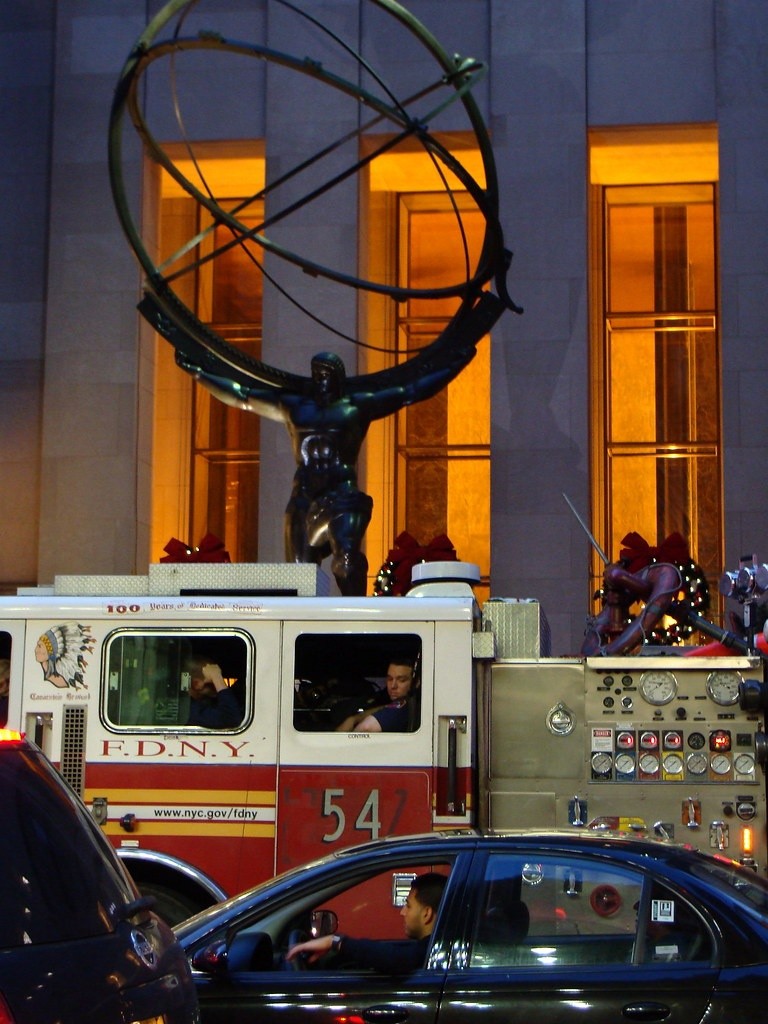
[331,934,346,952]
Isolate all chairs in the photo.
[482,902,529,946]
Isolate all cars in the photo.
[172,828,768,1024]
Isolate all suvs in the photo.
[0,727,202,1024]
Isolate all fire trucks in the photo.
[0,560,767,943]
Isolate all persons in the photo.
[186,656,244,728]
[174,346,479,596]
[334,658,420,732]
[628,901,681,962]
[287,872,448,972]
